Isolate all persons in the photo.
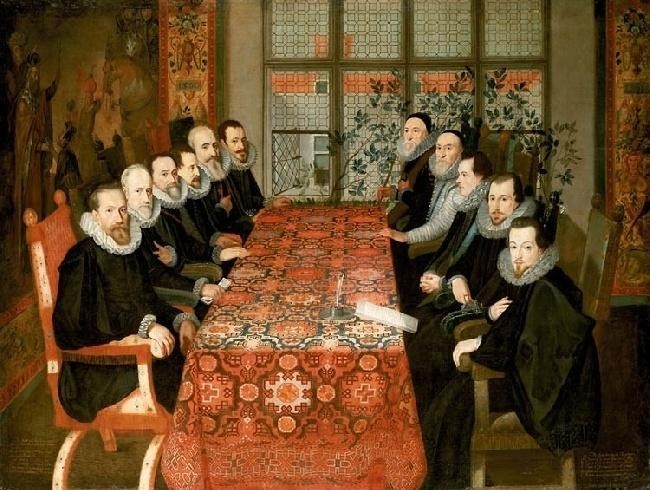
[13,38,60,279]
[375,112,603,489]
[53,118,291,425]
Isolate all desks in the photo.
[159,202,427,490]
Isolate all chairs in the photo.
[20,133,224,490]
[376,121,624,489]
